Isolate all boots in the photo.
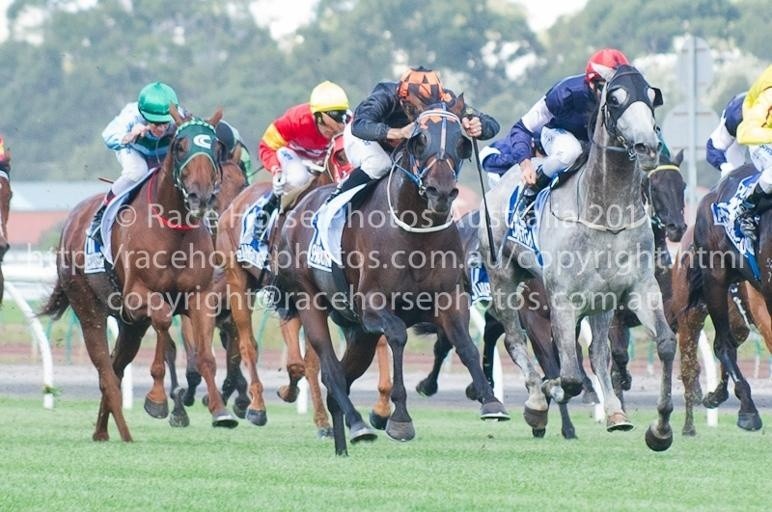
[327,167,377,221]
[255,192,281,243]
[736,183,772,240]
[518,163,553,225]
[90,187,117,246]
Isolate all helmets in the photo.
[138,82,178,123]
[311,81,349,114]
[397,66,455,123]
[586,49,630,82]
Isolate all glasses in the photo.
[317,111,351,126]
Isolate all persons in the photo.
[479,134,545,188]
[734,65,771,217]
[311,64,499,225]
[86,81,185,245]
[706,91,746,183]
[510,49,630,230]
[654,122,669,162]
[213,120,250,187]
[240,80,354,247]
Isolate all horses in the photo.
[25,100,239,443]
[681,160,772,432]
[576,148,687,415]
[266,92,511,458]
[159,140,252,430]
[0,134,14,312]
[476,62,678,453]
[213,110,394,440]
[671,221,772,437]
[413,209,601,440]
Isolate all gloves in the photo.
[272,172,286,197]
